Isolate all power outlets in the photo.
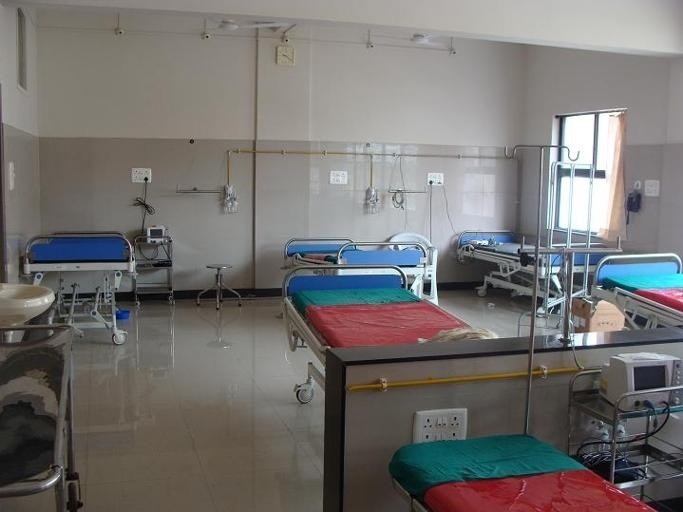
[414,407,468,443]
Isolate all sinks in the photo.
[0,282,55,327]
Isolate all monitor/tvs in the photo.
[599,354,683,411]
[147,227,165,244]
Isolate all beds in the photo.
[283,237,438,307]
[22,231,135,346]
[280,268,497,404]
[591,251,683,336]
[452,230,614,320]
[391,434,660,512]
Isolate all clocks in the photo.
[277,45,295,67]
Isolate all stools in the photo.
[198,310,239,352]
[197,263,242,311]
